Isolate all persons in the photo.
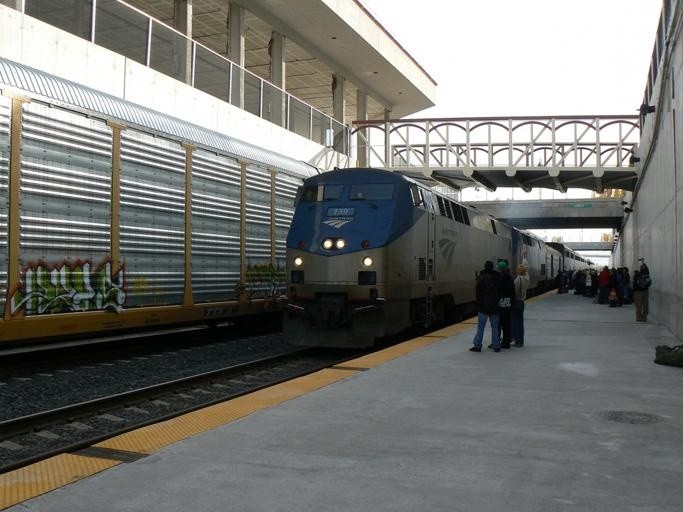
[469,249,531,352]
[553,257,630,307]
[631,263,650,321]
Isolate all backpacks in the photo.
[637,274,652,289]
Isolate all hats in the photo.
[495,262,507,270]
[517,264,529,273]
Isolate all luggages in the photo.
[608,288,618,307]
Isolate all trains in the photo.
[275,167,601,350]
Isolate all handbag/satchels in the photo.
[653,344,683,368]
[511,298,524,312]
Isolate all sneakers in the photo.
[488,339,524,352]
[469,346,481,352]
